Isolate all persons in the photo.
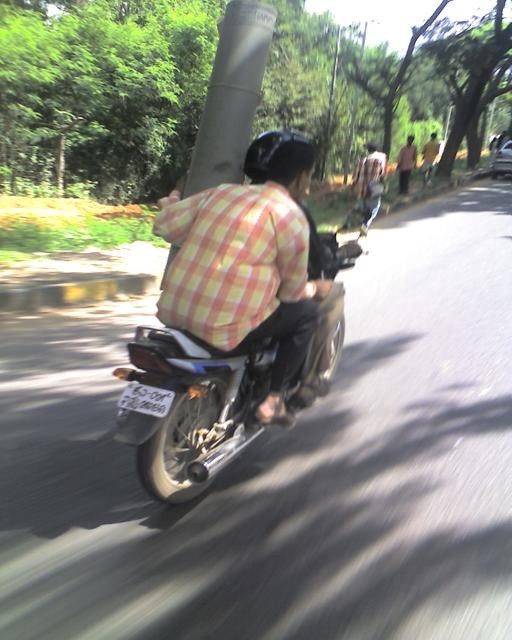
[237,127,363,410]
[497,129,506,152]
[392,134,418,198]
[487,137,497,169]
[151,130,339,430]
[420,132,441,191]
[348,139,388,229]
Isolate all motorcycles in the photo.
[112,180,391,504]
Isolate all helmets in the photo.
[245,131,285,180]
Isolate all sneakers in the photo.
[297,379,329,406]
[256,404,295,429]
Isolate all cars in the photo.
[490,134,512,182]
